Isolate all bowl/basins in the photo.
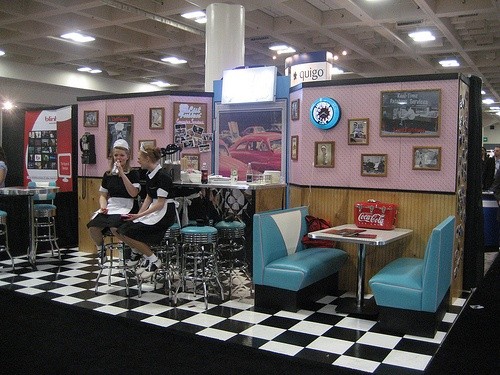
[190,175,201,182]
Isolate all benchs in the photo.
[368,216,455,338]
[252,205,348,312]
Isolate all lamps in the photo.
[309,97,341,130]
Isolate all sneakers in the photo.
[141,258,162,279]
[126,251,144,267]
[97,244,107,264]
[135,258,150,275]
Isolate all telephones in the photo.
[80,134,96,164]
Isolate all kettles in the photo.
[158,144,182,168]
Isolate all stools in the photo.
[93,219,253,311]
[0,210,16,269]
[29,203,61,263]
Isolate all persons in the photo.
[482,147,488,191]
[485,146,500,190]
[492,167,500,208]
[0,146,7,187]
[318,145,329,166]
[87,139,141,266]
[403,106,416,121]
[116,142,176,279]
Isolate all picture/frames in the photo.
[139,139,156,149]
[149,107,164,131]
[379,88,443,138]
[313,140,335,169]
[346,118,369,146]
[182,153,200,171]
[361,153,388,178]
[291,135,297,160]
[291,99,299,120]
[105,114,134,161]
[411,146,441,171]
[84,110,99,127]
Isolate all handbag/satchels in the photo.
[301,214,334,249]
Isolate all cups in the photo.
[253,173,272,185]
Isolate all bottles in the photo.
[112,160,120,175]
[201,162,209,184]
[246,162,253,186]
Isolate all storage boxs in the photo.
[353,198,399,230]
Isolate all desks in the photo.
[142,178,285,191]
[0,186,60,271]
[308,223,413,318]
